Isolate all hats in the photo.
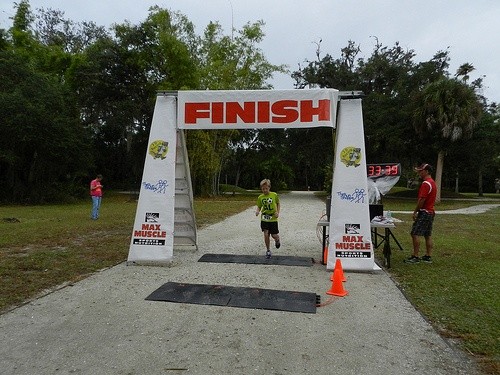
[416,164,433,172]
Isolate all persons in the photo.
[403,163,437,265]
[256,179,280,260]
[90,174,103,220]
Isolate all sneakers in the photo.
[275,235,280,249]
[265,250,271,259]
[407,258,421,264]
[419,255,432,263]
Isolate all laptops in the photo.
[370,204,383,221]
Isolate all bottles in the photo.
[386,209,391,221]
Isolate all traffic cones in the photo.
[330,259,347,282]
[325,269,349,297]
[323,246,328,265]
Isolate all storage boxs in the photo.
[369,204,383,222]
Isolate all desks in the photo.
[318,217,406,269]
